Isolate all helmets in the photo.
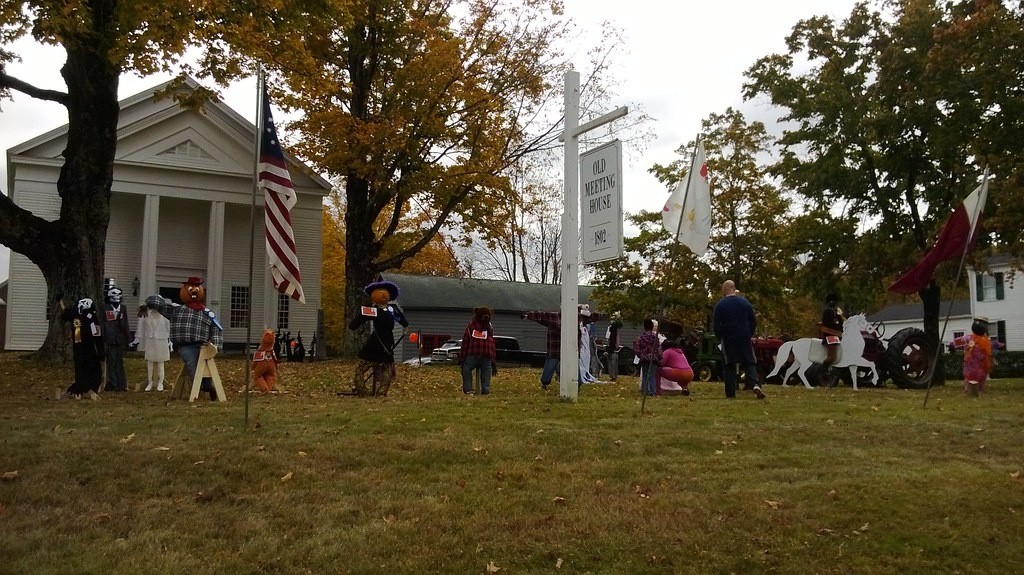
[826,293,840,303]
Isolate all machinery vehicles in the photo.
[794,318,937,391]
[689,329,788,382]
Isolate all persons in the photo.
[714,280,766,399]
[634,319,693,399]
[131,294,173,391]
[100,286,130,392]
[820,294,845,375]
[66,296,105,400]
[349,274,410,397]
[145,276,223,401]
[949,316,1005,397]
[521,304,623,390]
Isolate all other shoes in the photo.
[753,386,766,399]
[682,388,690,396]
[650,392,657,397]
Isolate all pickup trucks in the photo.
[431,334,548,369]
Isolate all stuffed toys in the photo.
[253,328,279,394]
[460,306,497,395]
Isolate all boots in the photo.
[817,360,832,377]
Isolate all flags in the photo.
[662,138,711,256]
[889,169,990,294]
[257,70,306,306]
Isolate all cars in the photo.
[409,341,466,368]
